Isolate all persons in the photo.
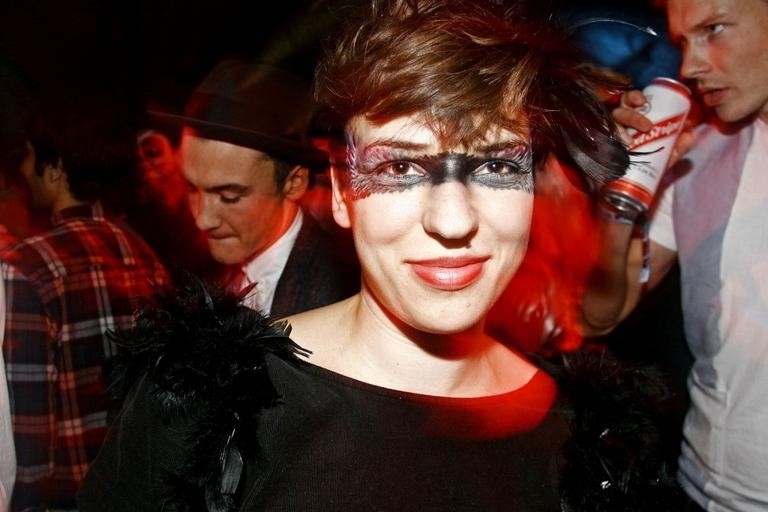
[578,2,768,511]
[298,110,347,235]
[131,89,211,274]
[0,98,175,512]
[68,0,708,511]
[146,57,348,321]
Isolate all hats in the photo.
[147,59,328,170]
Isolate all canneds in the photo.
[606,77,691,216]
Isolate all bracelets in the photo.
[594,200,637,226]
